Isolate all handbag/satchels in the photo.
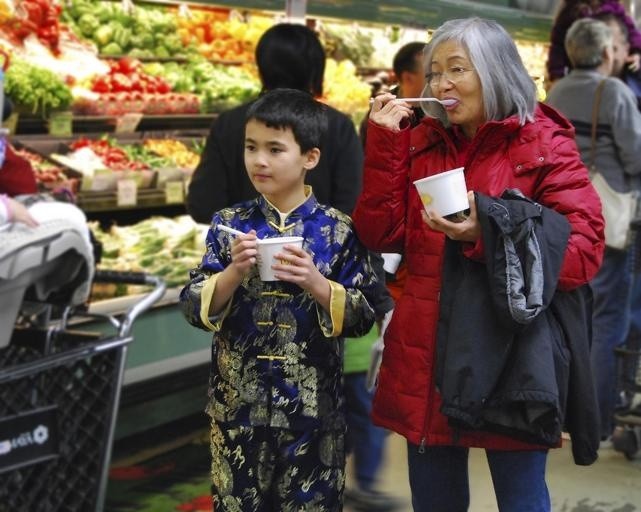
[587,76,637,252]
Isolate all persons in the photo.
[356,39,431,151]
[339,318,405,509]
[0,193,44,230]
[351,15,605,512]
[542,18,641,453]
[178,89,399,510]
[185,19,367,229]
[542,0,641,100]
[593,8,641,423]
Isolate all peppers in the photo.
[64,54,261,116]
[0,0,275,61]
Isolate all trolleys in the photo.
[0,269,168,512]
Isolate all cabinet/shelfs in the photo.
[0,1,555,449]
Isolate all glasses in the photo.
[424,65,474,88]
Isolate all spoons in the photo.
[369,96,458,106]
